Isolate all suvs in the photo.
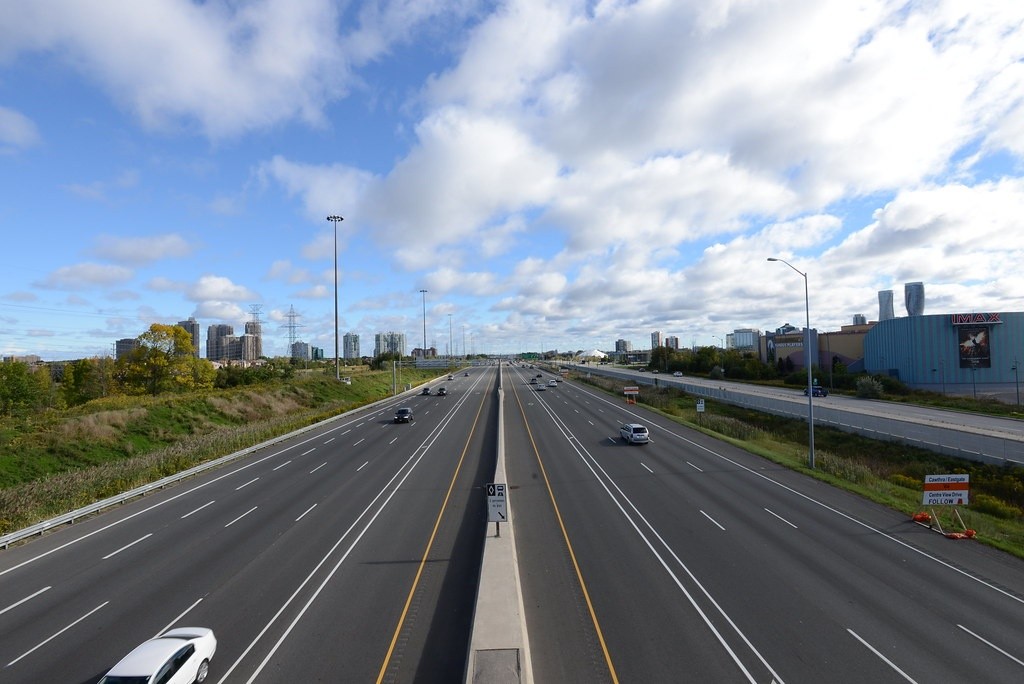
[620,423,650,445]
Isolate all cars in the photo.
[673,371,683,377]
[639,368,645,371]
[93,627,218,683]
[504,358,563,391]
[421,371,470,395]
[394,408,416,424]
[652,370,658,374]
[804,386,828,398]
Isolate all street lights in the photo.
[326,214,346,381]
[417,288,477,358]
[712,335,724,375]
[767,250,818,467]
